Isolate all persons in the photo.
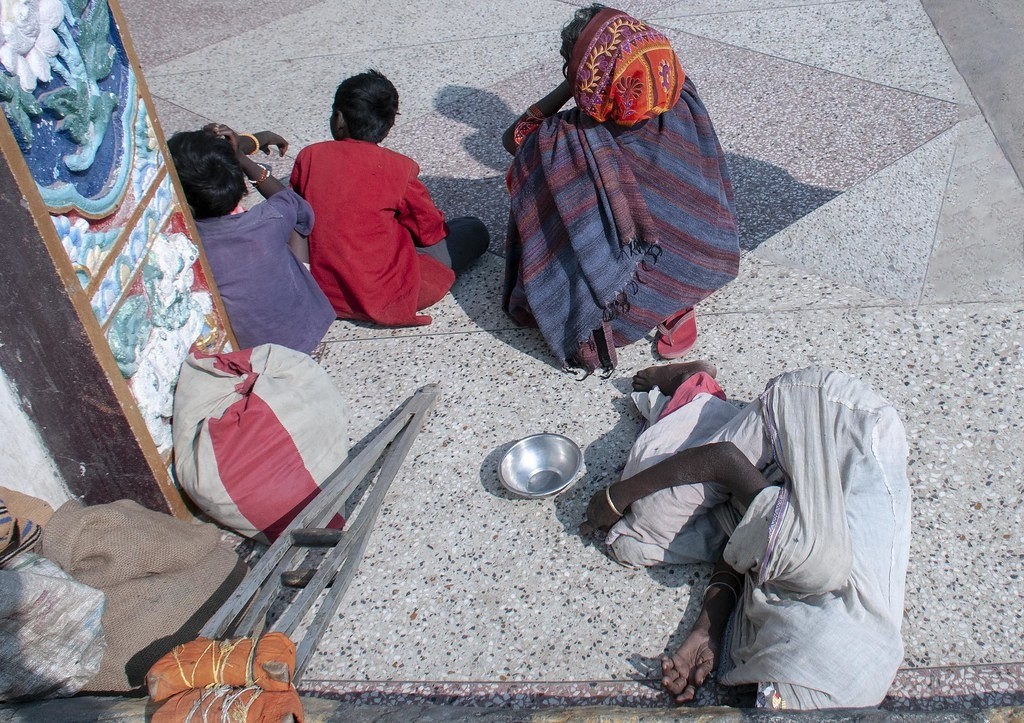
[501,2,740,381]
[579,360,912,709]
[166,123,337,354]
[288,68,491,327]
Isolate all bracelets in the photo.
[709,571,741,591]
[249,163,270,184]
[606,486,624,516]
[526,104,545,118]
[703,582,737,603]
[238,133,259,154]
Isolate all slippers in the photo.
[656,306,697,359]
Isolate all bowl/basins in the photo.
[498,433,582,499]
